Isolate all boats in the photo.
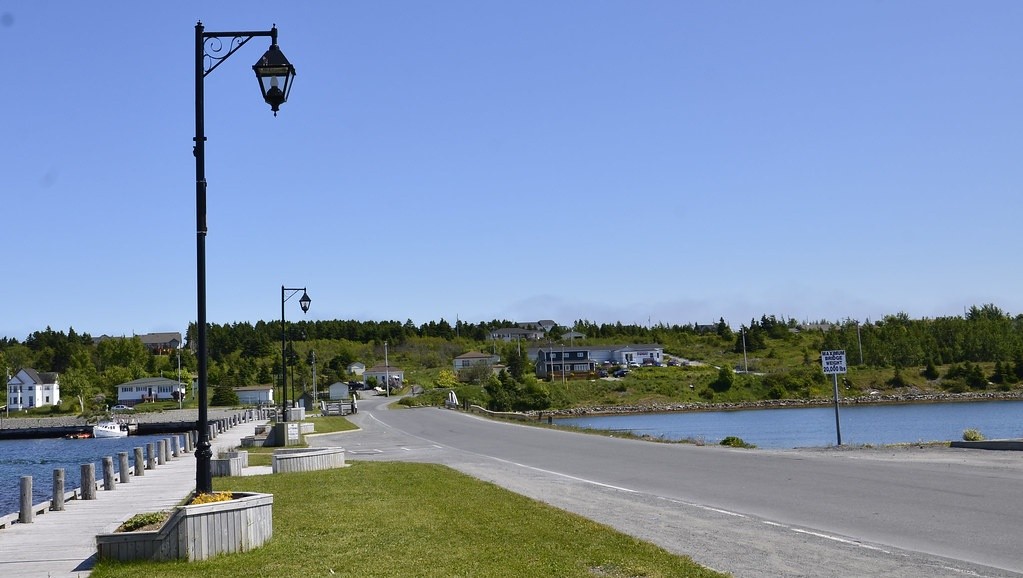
[65,430,93,439]
[93,421,129,438]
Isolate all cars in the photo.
[111,404,135,411]
[613,370,631,378]
[627,361,639,370]
[652,362,663,367]
[681,361,690,366]
[667,362,679,367]
[598,370,610,378]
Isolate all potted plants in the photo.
[211,445,248,477]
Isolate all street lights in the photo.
[561,344,567,383]
[288,327,308,408]
[177,352,182,409]
[742,328,748,374]
[185,19,301,492]
[856,319,863,364]
[384,341,391,399]
[279,284,312,422]
